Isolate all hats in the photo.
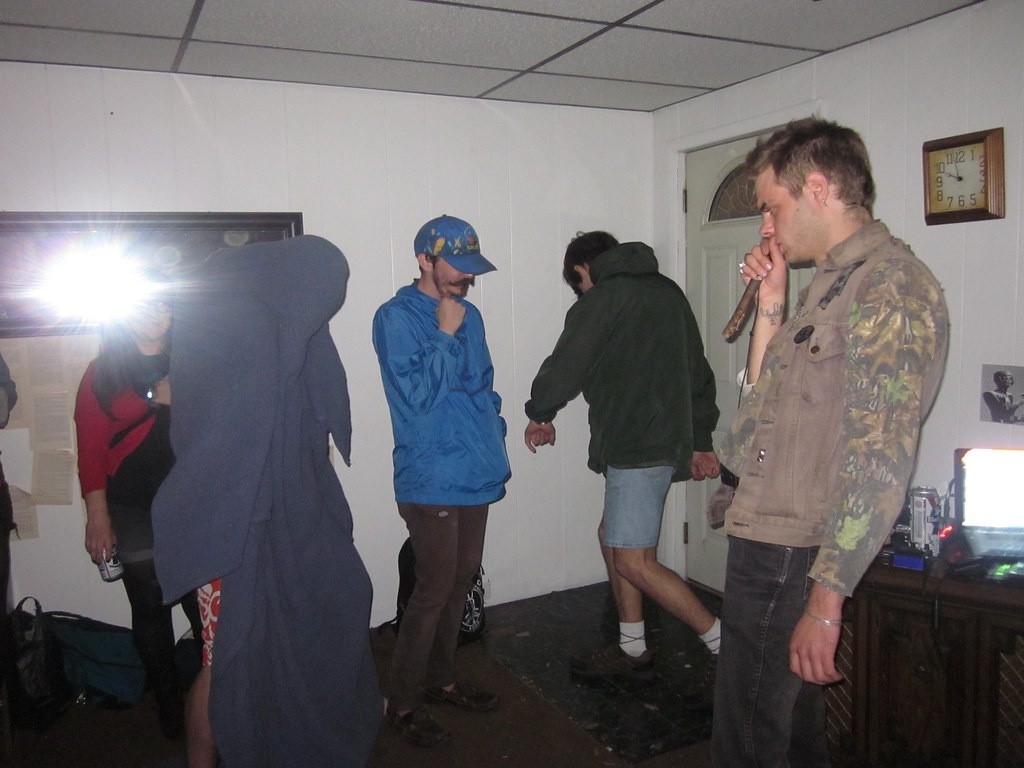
[414,214,497,275]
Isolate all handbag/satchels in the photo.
[1,596,79,728]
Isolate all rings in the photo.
[737,260,747,274]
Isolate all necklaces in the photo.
[141,367,161,401]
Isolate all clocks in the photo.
[923,126,1007,226]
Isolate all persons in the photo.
[74,269,204,736]
[710,115,950,768]
[526,229,725,681]
[372,213,514,747]
[0,346,21,709]
[983,370,1024,424]
[182,576,220,767]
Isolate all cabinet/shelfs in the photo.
[824,565,1024,768]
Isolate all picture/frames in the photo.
[0,212,303,339]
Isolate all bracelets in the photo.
[805,606,844,628]
[534,420,550,426]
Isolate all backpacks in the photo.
[377,533,485,643]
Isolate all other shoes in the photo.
[156,690,187,741]
[568,643,656,681]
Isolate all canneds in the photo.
[97,544,124,582]
[909,486,942,556]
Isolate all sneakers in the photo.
[387,704,450,748]
[673,647,717,707]
[424,678,501,711]
